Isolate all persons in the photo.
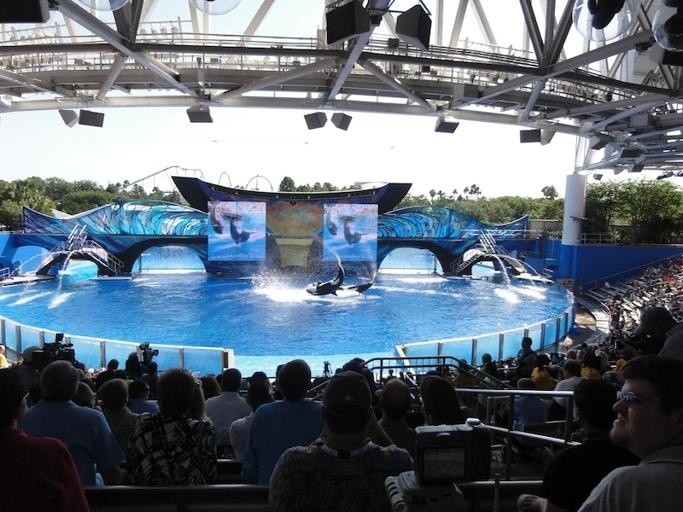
[22,360,272,458]
[241,361,324,485]
[599,252,682,337]
[542,379,642,510]
[517,355,683,510]
[375,308,683,451]
[1,366,89,509]
[20,360,125,487]
[269,370,400,510]
[120,369,217,487]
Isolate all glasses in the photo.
[617,392,646,405]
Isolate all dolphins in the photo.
[336,213,366,246]
[209,203,224,235]
[222,212,255,246]
[326,209,337,237]
[306,266,344,297]
[340,283,373,295]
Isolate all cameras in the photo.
[416,418,492,485]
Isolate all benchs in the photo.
[584,257,682,320]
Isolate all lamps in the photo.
[304,111,328,130]
[186,106,213,125]
[58,109,105,128]
[652,1,682,53]
[434,114,459,134]
[325,0,433,52]
[518,124,555,145]
[79,1,132,13]
[587,109,681,182]
[571,1,644,42]
[78,453,550,512]
[331,113,352,131]
[1,1,60,24]
[189,0,241,15]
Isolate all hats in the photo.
[276,360,412,422]
[630,307,673,337]
